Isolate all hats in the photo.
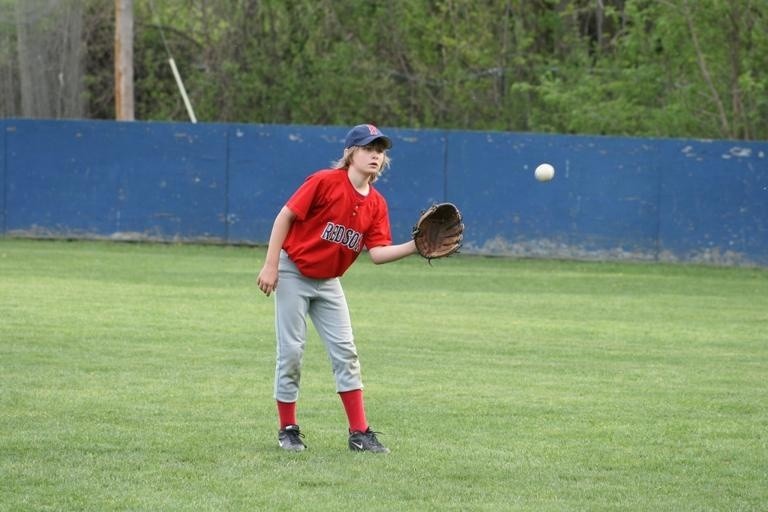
[345,124,392,150]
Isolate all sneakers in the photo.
[278,424,307,451]
[348,425,391,454]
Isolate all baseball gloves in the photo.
[414,201,465,259]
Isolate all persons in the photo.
[257,124,464,454]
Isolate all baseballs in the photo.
[534,163,555,184]
